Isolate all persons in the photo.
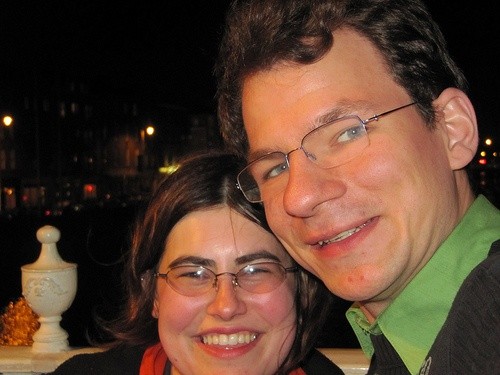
[215,0,500,373]
[50,152,349,375]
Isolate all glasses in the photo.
[236,98,424,203]
[149,261,300,297]
